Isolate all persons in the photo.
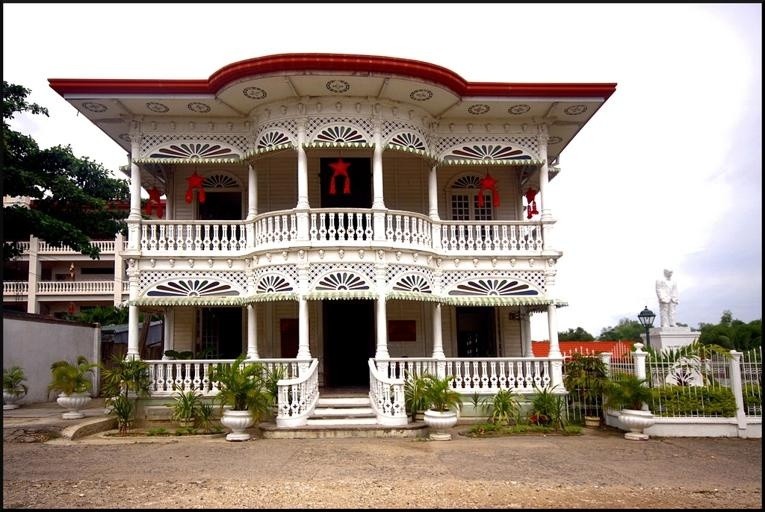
[656,267,677,329]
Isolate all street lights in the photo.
[637,306,656,388]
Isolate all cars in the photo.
[540,360,605,415]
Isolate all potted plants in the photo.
[205,350,276,442]
[416,373,463,443]
[46,356,104,419]
[2,365,30,411]
[602,372,660,441]
